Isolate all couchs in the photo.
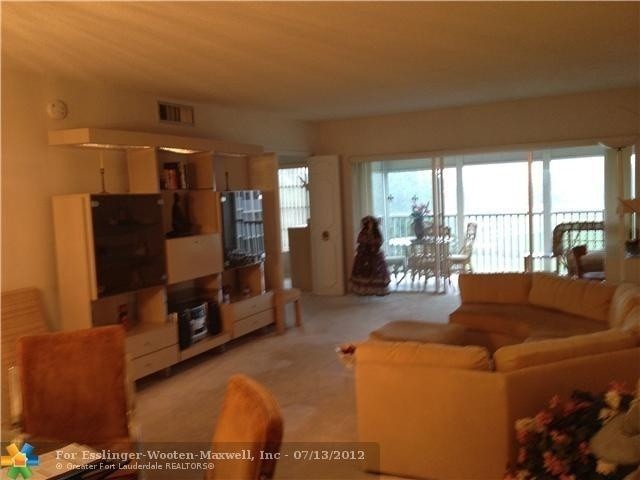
[356,273,640,480]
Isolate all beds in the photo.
[369,319,460,344]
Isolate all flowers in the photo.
[409,195,430,224]
[504,378,640,480]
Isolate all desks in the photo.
[0,434,130,480]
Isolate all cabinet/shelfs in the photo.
[49,127,301,384]
[288,225,312,291]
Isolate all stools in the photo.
[524,253,560,274]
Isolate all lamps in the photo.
[600,132,637,281]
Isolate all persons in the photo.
[170,191,189,230]
[347,215,392,296]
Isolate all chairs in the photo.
[385,222,476,294]
[18,325,139,480]
[205,373,284,480]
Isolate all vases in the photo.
[414,220,424,237]
